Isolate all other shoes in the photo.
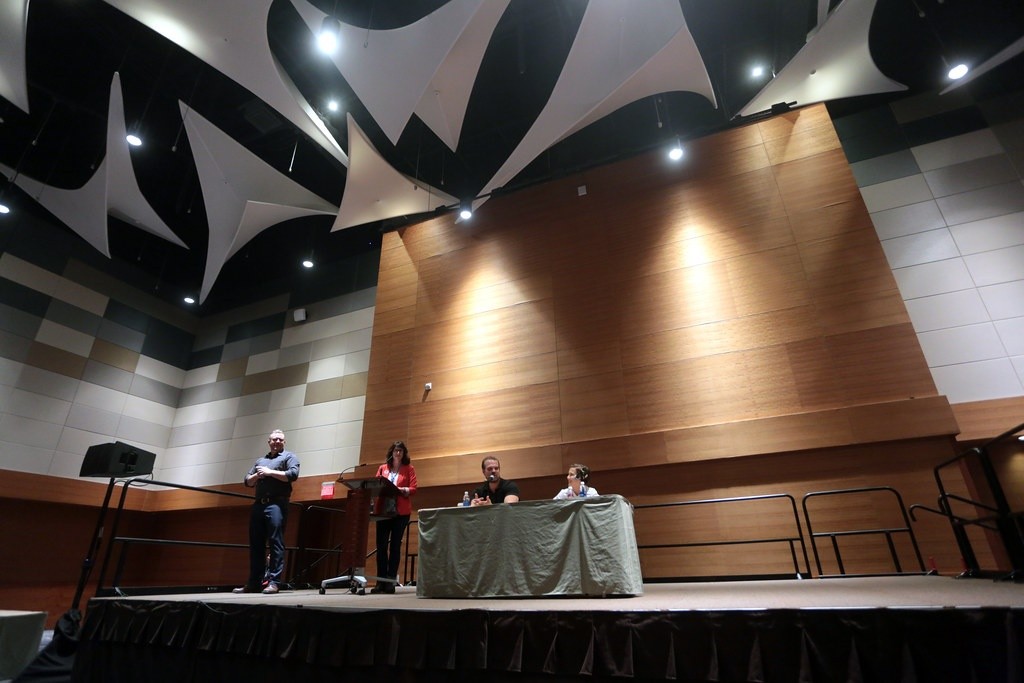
[370,581,395,593]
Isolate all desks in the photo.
[1,610,47,680]
[419,494,643,598]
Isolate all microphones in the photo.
[338,463,368,479]
[487,475,494,483]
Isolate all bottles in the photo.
[463,491,470,506]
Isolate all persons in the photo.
[233,429,300,593]
[551,463,600,500]
[369,441,417,593]
[460,456,520,505]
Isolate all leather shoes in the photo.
[231,582,260,593]
[262,583,279,593]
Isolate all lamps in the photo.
[942,49,969,78]
[669,136,683,160]
[460,196,473,219]
[0,193,14,213]
[302,250,315,268]
[183,288,195,304]
[125,120,148,146]
